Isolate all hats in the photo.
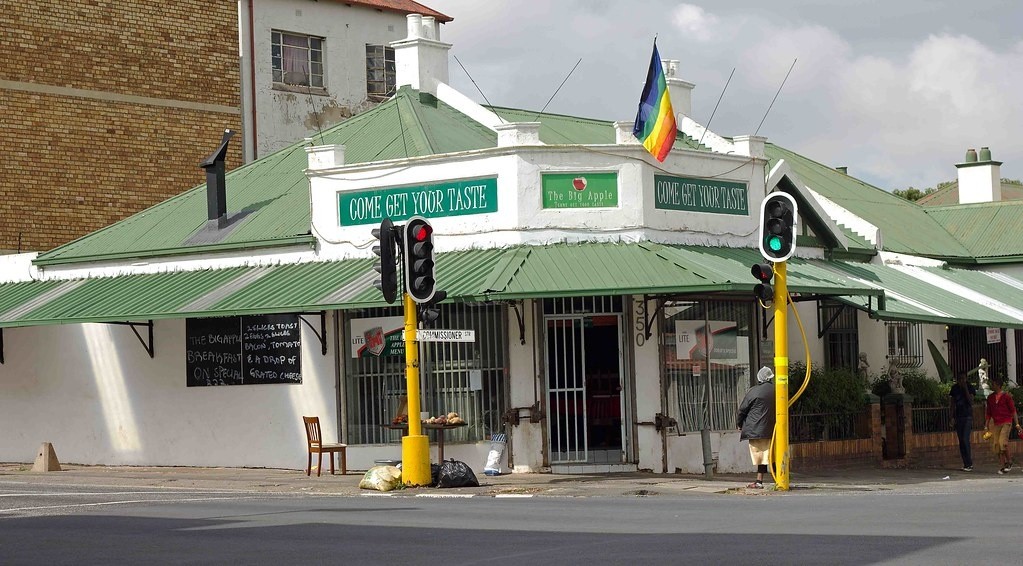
[757,366,774,383]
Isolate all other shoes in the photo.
[747,483,763,489]
[1003,463,1013,471]
[997,468,1006,474]
[961,466,974,471]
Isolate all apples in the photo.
[392,414,408,424]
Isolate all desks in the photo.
[380,422,468,464]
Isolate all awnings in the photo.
[0,243,889,366]
[786,254,1023,340]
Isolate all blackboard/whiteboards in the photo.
[186,313,303,388]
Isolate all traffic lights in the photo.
[760,193,797,262]
[404,216,436,303]
[372,218,397,304]
[751,263,773,301]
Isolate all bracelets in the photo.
[1016,424,1020,427]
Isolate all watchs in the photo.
[984,426,987,429]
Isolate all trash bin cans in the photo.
[374,459,397,466]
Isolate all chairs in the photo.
[303,416,348,476]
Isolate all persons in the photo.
[983,379,1023,475]
[857,352,870,392]
[888,358,904,388]
[736,365,775,489]
[948,371,977,472]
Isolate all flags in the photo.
[633,38,677,162]
